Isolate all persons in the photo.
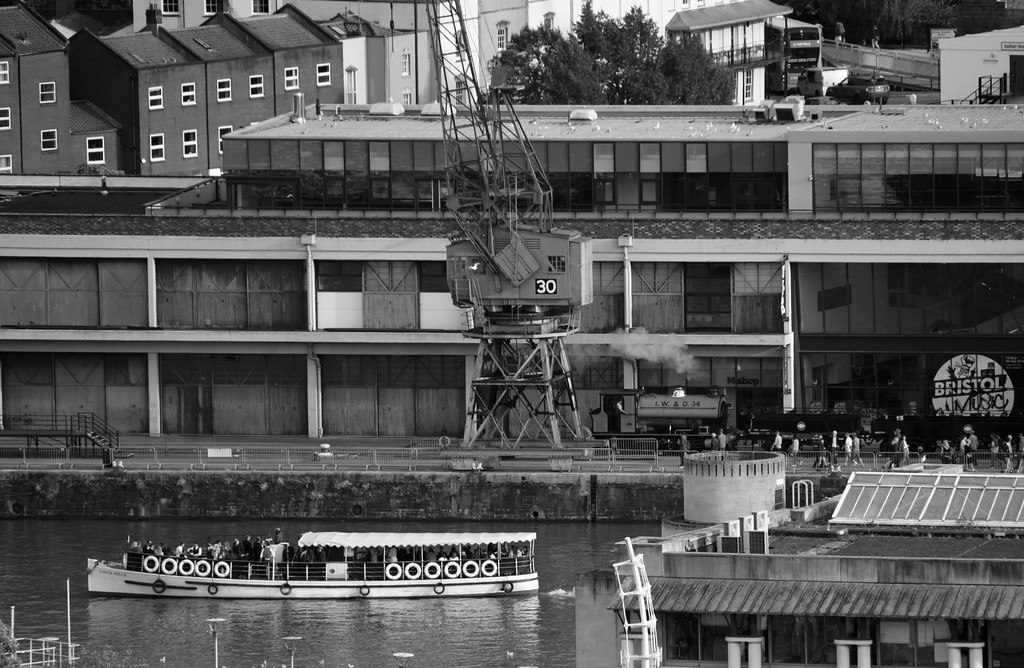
[129,528,531,581]
[675,424,1024,474]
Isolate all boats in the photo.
[86,530,540,600]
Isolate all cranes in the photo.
[425,1,597,448]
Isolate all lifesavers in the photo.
[143,555,159,573]
[502,581,513,593]
[153,580,166,594]
[161,558,177,575]
[359,586,370,596]
[213,561,230,578]
[280,583,292,595]
[481,559,497,577]
[405,563,422,580]
[439,436,451,448]
[386,563,403,580]
[434,583,445,594]
[179,559,194,576]
[444,561,461,579]
[424,562,441,579]
[194,560,211,577]
[463,560,480,578]
[208,584,218,595]
